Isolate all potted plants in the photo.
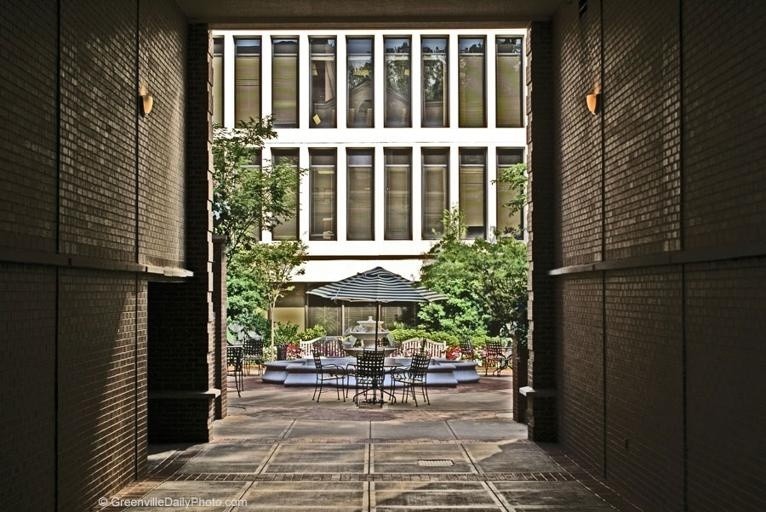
[274,335,289,359]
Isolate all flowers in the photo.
[445,346,461,360]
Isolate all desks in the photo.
[491,346,514,375]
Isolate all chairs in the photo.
[226,339,264,397]
[310,349,435,407]
[485,342,502,376]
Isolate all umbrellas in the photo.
[305,267,449,368]
